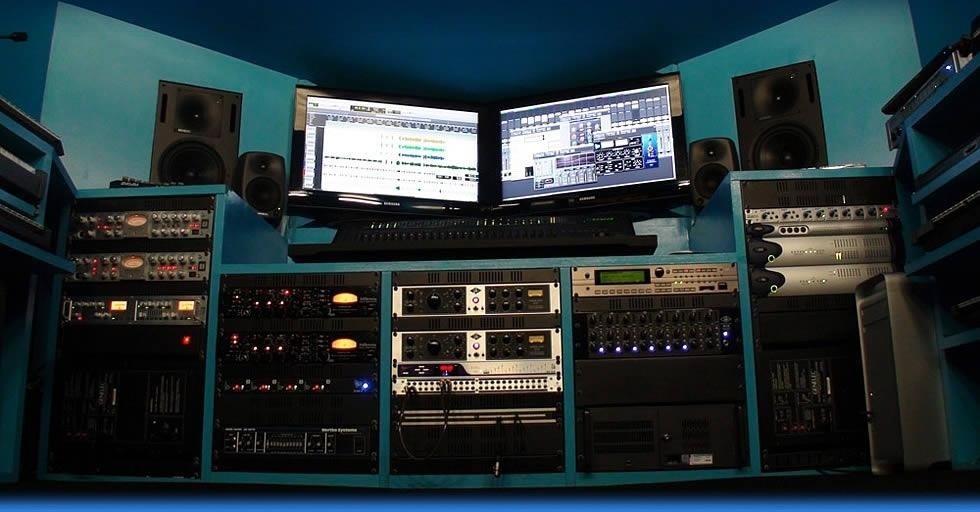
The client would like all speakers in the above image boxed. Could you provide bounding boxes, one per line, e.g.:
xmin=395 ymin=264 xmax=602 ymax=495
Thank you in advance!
xmin=689 ymin=137 xmax=739 ymax=211
xmin=148 ymin=79 xmax=242 ymax=196
xmin=732 ymin=59 xmax=829 ymax=172
xmin=237 ymin=151 xmax=286 ymax=224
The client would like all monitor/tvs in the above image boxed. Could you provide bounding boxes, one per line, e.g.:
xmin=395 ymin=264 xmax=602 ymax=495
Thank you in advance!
xmin=492 ymin=82 xmax=678 ymax=212
xmin=302 ymin=93 xmax=491 ymax=212
